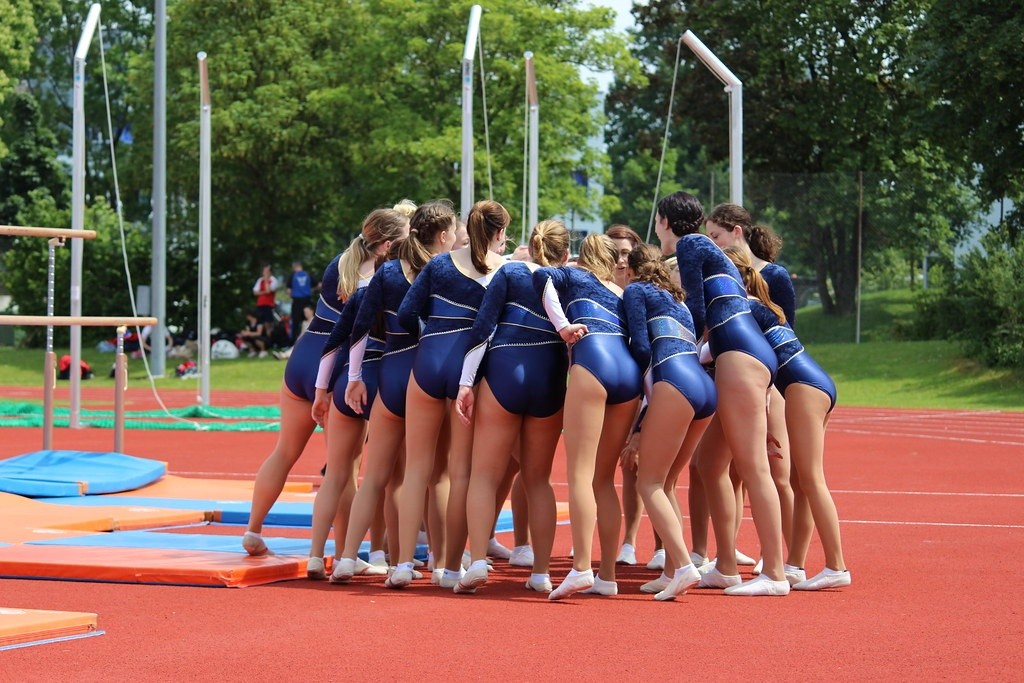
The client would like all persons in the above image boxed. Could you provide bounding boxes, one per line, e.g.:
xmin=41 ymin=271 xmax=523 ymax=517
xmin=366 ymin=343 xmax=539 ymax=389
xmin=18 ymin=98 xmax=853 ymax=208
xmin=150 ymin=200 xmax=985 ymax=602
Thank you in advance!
xmin=59 ymin=319 xmax=200 ymax=382
xmin=243 ymin=191 xmax=851 ymax=600
xmin=237 ymin=262 xmax=322 ymax=360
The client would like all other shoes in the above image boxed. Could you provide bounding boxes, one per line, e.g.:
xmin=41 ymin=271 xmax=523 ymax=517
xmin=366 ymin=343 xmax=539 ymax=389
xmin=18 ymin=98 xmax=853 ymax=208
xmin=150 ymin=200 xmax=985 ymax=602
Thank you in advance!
xmin=575 ymin=577 xmax=617 ymax=596
xmin=724 ymin=576 xmax=790 ymax=595
xmin=368 ymin=557 xmax=390 ymax=572
xmin=616 ymin=544 xmax=637 ymax=565
xmin=647 ymin=548 xmax=665 ymax=569
xmin=487 ymin=539 xmax=511 ymax=558
xmin=639 ymin=575 xmax=689 ymax=595
xmin=258 ymin=352 xmax=267 ymax=359
xmin=754 ymin=558 xmax=763 ymax=573
xmin=785 ymin=568 xmax=806 ymax=586
xmin=331 ymin=557 xmax=386 ymax=575
xmin=307 ymin=558 xmax=325 ymax=578
xmin=735 ymin=548 xmax=755 ymax=565
xmin=654 ymin=565 xmax=701 ymax=600
xmin=389 ymin=567 xmax=421 ymax=578
xmin=247 ymin=351 xmax=254 ymax=357
xmin=547 ymin=570 xmax=594 ymax=599
xmin=330 ymin=559 xmax=354 ymax=583
xmin=508 ymin=551 xmax=534 ymax=566
xmin=385 ymin=553 xmax=424 ymax=567
xmin=690 ymin=553 xmax=710 ymax=568
xmin=384 ymin=568 xmax=409 ymax=587
xmin=427 ymin=551 xmax=495 ymax=593
xmin=273 ymin=351 xmax=286 ymax=360
xmin=526 ymin=579 xmax=552 ymax=591
xmin=694 ymin=557 xmax=742 ymax=588
xmin=791 ymin=568 xmax=852 ymax=591
xmin=242 ymin=536 xmax=275 ymax=556
